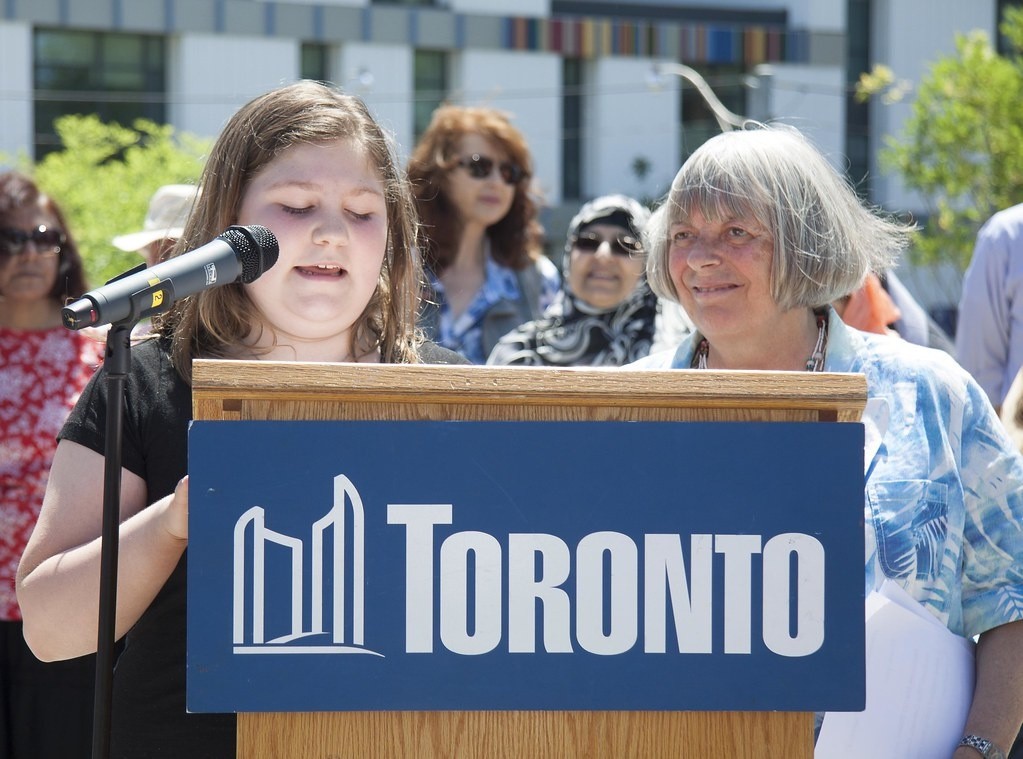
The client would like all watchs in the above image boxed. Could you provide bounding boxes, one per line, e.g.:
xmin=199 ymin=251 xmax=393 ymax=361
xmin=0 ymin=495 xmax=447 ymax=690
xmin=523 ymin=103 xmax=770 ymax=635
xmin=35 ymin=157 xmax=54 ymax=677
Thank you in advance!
xmin=957 ymin=735 xmax=1006 ymax=759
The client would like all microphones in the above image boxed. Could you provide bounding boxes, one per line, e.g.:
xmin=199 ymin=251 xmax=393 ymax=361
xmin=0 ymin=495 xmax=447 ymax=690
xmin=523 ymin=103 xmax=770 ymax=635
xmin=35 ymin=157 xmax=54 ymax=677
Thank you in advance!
xmin=61 ymin=224 xmax=279 ymax=330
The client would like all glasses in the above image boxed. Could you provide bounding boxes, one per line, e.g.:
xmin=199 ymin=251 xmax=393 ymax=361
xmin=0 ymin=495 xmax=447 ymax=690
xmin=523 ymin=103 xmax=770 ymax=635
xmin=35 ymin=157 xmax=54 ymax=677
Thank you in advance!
xmin=0 ymin=227 xmax=63 ymax=256
xmin=442 ymin=156 xmax=522 ymax=185
xmin=576 ymin=236 xmax=637 ymax=257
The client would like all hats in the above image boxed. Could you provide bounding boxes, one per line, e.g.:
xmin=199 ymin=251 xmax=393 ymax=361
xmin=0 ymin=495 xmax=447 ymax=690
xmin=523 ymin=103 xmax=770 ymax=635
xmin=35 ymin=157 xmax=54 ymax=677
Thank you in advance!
xmin=113 ymin=184 xmax=203 ymax=252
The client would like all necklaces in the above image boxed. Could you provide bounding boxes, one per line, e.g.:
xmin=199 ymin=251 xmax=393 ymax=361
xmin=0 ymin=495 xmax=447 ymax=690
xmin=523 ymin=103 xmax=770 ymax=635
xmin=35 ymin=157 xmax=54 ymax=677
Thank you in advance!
xmin=697 ymin=307 xmax=826 ymax=372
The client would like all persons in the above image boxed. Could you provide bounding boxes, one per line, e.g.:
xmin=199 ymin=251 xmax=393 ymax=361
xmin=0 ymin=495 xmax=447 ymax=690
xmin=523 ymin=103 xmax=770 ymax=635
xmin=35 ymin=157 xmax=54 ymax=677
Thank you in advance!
xmin=617 ymin=129 xmax=1023 ymax=759
xmin=484 ymin=194 xmax=696 ymax=368
xmin=956 ymin=203 xmax=1023 ymax=417
xmin=13 ymin=80 xmax=420 ymax=759
xmin=406 ymin=103 xmax=562 ymax=363
xmin=831 ymin=252 xmax=953 ymax=355
xmin=0 ymin=168 xmax=204 ymax=759
